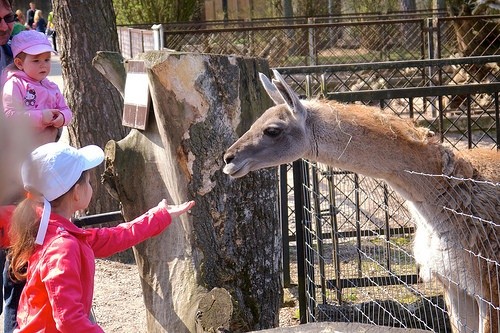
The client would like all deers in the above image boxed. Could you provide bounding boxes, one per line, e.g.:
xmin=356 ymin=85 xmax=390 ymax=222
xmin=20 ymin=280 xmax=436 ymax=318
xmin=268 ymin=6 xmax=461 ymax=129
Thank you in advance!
xmin=223 ymin=68 xmax=500 ymax=333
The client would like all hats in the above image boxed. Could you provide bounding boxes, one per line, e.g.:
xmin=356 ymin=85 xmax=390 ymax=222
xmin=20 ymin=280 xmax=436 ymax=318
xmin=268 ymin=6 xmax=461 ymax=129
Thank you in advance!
xmin=11 ymin=30 xmax=59 ymax=59
xmin=21 ymin=142 xmax=106 ymax=203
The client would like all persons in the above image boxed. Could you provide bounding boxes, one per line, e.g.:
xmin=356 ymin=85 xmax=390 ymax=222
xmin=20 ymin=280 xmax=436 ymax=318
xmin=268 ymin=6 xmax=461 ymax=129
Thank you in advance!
xmin=27 ymin=2 xmax=36 ymax=28
xmin=15 ymin=9 xmax=25 ymax=26
xmin=46 ymin=10 xmax=57 ymax=52
xmin=0 ymin=26 xmax=72 ymax=134
xmin=0 ymin=0 xmax=62 ymax=333
xmin=33 ymin=10 xmax=47 ymax=33
xmin=0 ymin=141 xmax=196 ymax=333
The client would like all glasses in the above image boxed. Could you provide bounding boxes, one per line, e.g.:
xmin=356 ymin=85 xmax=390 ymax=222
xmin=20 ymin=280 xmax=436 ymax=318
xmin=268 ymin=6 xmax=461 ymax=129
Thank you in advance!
xmin=0 ymin=12 xmax=15 ymax=24
